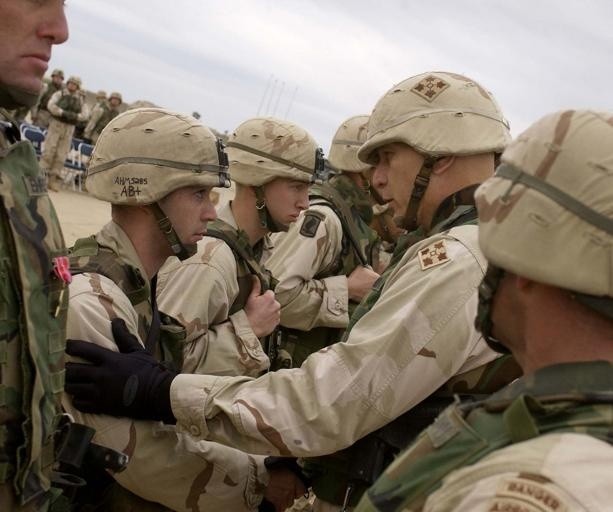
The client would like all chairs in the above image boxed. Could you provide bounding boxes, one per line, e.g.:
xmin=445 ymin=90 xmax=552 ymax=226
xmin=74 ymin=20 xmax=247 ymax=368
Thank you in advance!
xmin=78 ymin=143 xmax=95 ymax=193
xmin=20 ymin=123 xmax=42 ymax=155
xmin=60 ymin=158 xmax=74 ymax=190
xmin=73 ymin=137 xmax=93 ymax=189
xmin=23 ymin=129 xmax=45 ymax=159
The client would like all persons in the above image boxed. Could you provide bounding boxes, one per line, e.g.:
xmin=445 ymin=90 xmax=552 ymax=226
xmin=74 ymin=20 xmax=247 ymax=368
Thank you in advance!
xmin=64 ymin=106 xmax=304 ymax=511
xmin=63 ymin=71 xmax=510 ymax=512
xmin=352 ymin=108 xmax=613 ymax=511
xmin=38 ymin=75 xmax=89 ymax=196
xmin=261 ymin=115 xmax=381 ymax=330
xmin=0 ymin=0 xmax=69 ymax=512
xmin=31 ymin=69 xmax=122 ymax=144
xmin=157 ymin=116 xmax=316 ymax=377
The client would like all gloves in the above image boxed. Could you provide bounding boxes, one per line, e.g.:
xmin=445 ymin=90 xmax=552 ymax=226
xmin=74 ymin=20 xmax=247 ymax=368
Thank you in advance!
xmin=64 ymin=317 xmax=177 ymax=428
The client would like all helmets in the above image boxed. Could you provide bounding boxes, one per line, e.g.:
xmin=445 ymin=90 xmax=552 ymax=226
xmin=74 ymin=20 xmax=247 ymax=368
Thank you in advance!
xmin=86 ymin=108 xmax=232 ymax=206
xmin=51 ymin=69 xmax=64 ymax=80
xmin=222 ymin=117 xmax=317 ymax=187
xmin=108 ymin=92 xmax=122 ymax=103
xmin=96 ymin=90 xmax=107 ymax=98
xmin=66 ymin=74 xmax=82 ymax=89
xmin=473 ymin=109 xmax=613 ymax=298
xmin=356 ymin=69 xmax=513 ymax=166
xmin=327 ymin=115 xmax=369 ymax=173
